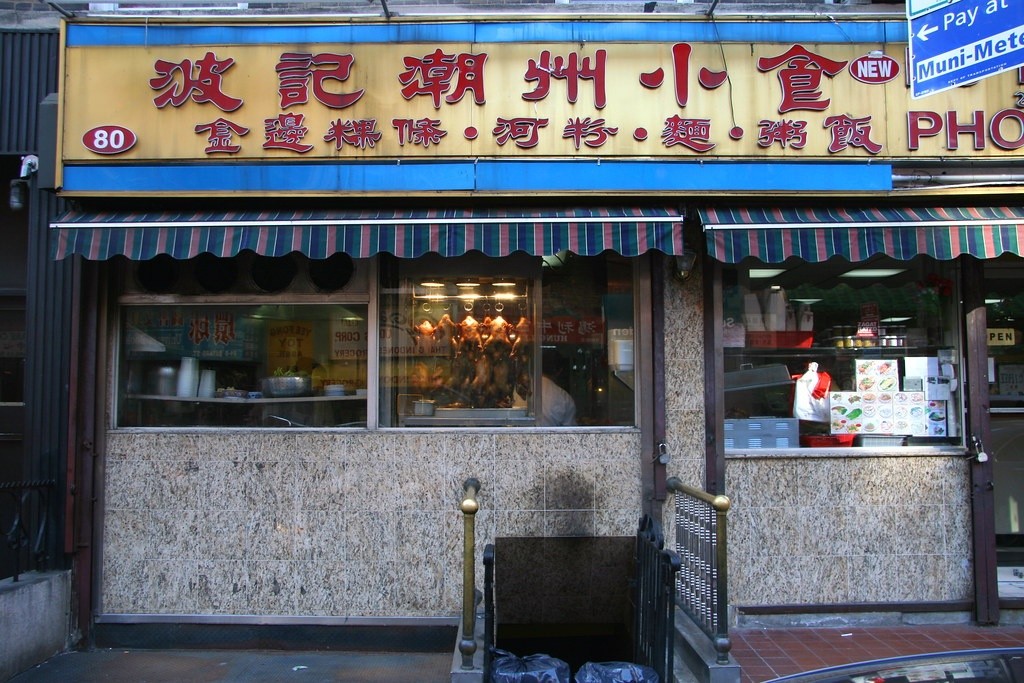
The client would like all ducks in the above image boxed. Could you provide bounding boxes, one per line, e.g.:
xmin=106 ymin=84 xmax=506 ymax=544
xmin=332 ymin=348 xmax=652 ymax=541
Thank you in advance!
xmin=413 ymin=315 xmax=532 ymax=357
xmin=410 ymin=355 xmax=531 ymax=401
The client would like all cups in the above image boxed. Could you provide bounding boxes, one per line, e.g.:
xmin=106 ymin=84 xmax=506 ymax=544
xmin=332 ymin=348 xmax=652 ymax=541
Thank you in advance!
xmin=744 ymin=293 xmax=764 ymax=331
xmin=198 ymin=370 xmax=215 ymax=397
xmin=175 ymin=356 xmax=198 ymax=397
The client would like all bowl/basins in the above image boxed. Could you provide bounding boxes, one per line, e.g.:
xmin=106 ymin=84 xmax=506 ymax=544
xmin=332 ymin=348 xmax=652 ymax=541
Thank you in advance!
xmin=214 ymin=391 xmax=223 ymax=398
xmin=259 ymin=376 xmax=311 ymax=398
xmin=323 ymin=384 xmax=345 ymax=396
xmin=223 ymin=390 xmax=247 ymax=397
xmin=355 ymin=389 xmax=367 ymax=395
xmin=248 ymin=392 xmax=262 ymax=399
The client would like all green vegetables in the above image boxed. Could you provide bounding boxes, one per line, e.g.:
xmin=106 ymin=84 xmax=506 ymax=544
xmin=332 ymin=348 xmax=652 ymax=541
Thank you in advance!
xmin=271 ymin=367 xmax=310 ymax=377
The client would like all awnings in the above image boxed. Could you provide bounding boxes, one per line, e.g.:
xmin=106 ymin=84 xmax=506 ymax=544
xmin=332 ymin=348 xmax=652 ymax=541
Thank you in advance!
xmin=696 ymin=205 xmax=1024 ymax=264
xmin=49 ymin=212 xmax=684 ymax=260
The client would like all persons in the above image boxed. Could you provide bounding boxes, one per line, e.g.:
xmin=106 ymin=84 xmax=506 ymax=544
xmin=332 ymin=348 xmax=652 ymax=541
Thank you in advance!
xmin=804 ymin=358 xmax=843 ymax=433
xmin=506 ymin=350 xmax=576 ymax=426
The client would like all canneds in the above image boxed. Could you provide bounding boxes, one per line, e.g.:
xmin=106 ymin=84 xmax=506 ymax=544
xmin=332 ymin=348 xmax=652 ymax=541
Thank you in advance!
xmin=823 ymin=324 xmax=908 ymax=347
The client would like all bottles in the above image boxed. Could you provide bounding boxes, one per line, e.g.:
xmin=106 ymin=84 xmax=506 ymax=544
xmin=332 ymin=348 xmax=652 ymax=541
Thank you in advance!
xmin=765 ymin=286 xmax=786 ymax=331
